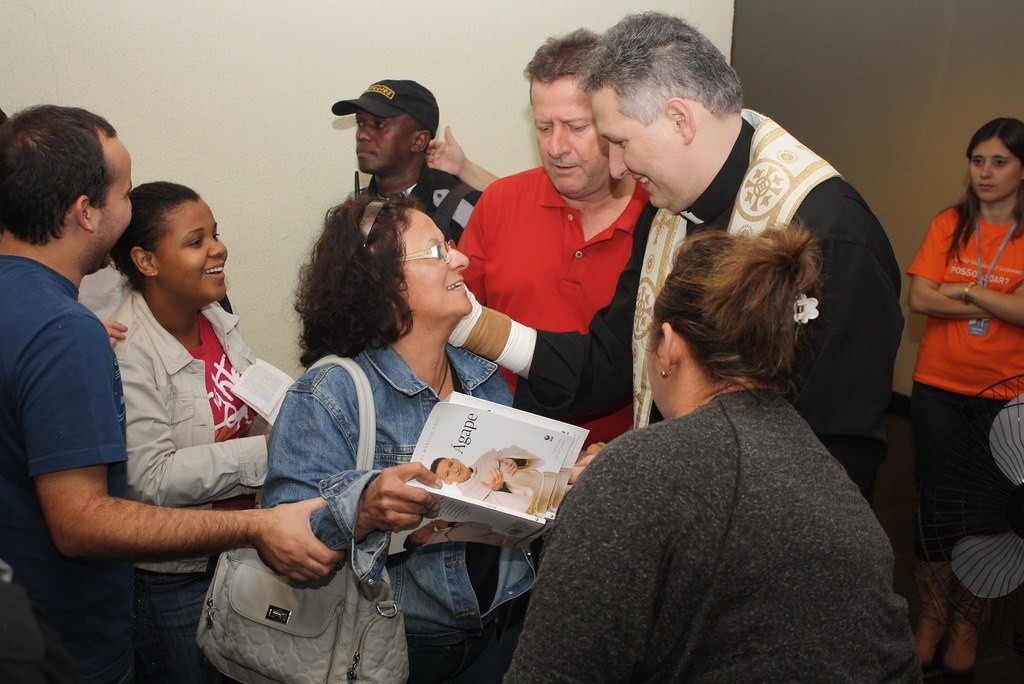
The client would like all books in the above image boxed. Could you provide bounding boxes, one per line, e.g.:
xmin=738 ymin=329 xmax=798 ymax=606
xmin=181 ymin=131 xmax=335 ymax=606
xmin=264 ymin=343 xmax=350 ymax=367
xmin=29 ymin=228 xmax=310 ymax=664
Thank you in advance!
xmin=233 ymin=360 xmax=295 ymax=427
xmin=389 ymin=390 xmax=590 ymax=556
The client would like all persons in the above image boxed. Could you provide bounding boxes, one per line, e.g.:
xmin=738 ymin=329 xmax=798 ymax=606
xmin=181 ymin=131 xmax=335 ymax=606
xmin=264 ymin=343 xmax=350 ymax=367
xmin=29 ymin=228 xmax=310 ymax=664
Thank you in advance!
xmin=259 ymin=200 xmax=607 ymax=684
xmin=904 ymin=118 xmax=1024 ymax=684
xmin=0 ymin=106 xmax=345 ymax=684
xmin=432 ymin=446 xmax=558 ymax=515
xmin=450 ymin=12 xmax=902 ymax=517
xmin=334 ymin=76 xmax=498 ymax=252
xmin=457 ymin=25 xmax=653 ymax=451
xmin=975 ymin=318 xmax=982 ymax=328
xmin=403 ymin=520 xmax=524 ymax=551
xmin=503 ymin=226 xmax=920 ymax=684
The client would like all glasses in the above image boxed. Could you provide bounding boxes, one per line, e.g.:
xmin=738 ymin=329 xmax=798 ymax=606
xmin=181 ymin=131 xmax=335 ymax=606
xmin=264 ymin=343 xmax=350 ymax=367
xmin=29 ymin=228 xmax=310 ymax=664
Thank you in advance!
xmin=396 ymin=239 xmax=456 ymax=265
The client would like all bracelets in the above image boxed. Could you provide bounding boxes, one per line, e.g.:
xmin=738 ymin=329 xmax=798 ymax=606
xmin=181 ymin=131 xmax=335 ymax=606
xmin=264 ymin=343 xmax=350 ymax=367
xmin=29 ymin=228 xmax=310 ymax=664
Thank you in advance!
xmin=963 ymin=282 xmax=975 ymax=304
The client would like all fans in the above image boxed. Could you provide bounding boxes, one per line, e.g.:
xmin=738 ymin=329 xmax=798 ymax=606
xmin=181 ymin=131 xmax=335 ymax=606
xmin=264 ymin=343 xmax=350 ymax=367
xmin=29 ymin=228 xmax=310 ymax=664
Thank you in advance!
xmin=917 ymin=374 xmax=1024 ymax=663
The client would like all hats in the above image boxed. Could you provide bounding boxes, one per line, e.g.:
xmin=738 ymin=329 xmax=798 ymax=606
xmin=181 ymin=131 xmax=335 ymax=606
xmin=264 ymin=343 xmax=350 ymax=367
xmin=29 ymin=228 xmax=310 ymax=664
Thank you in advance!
xmin=332 ymin=79 xmax=439 ymax=139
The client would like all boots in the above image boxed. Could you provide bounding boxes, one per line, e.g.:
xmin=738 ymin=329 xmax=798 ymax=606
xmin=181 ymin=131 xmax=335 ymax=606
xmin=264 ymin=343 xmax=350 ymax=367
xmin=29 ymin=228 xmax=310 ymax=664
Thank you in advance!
xmin=943 ymin=575 xmax=981 ymax=672
xmin=912 ymin=556 xmax=950 ymax=665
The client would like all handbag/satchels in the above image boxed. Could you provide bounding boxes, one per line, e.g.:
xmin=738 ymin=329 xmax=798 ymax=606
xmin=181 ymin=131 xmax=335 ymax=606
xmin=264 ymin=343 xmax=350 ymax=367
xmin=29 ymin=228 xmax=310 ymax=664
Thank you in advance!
xmin=194 ymin=355 xmax=409 ymax=684
